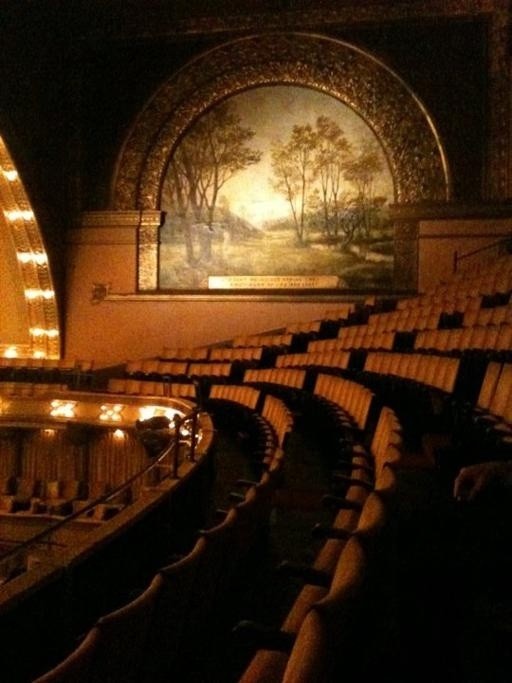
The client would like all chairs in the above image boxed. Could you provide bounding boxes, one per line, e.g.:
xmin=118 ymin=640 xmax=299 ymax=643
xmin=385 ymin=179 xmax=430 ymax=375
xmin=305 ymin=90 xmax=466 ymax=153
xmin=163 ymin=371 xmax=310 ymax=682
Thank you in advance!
xmin=231 ymin=252 xmax=512 ymax=350
xmin=0 ymin=346 xmax=512 ymax=683
